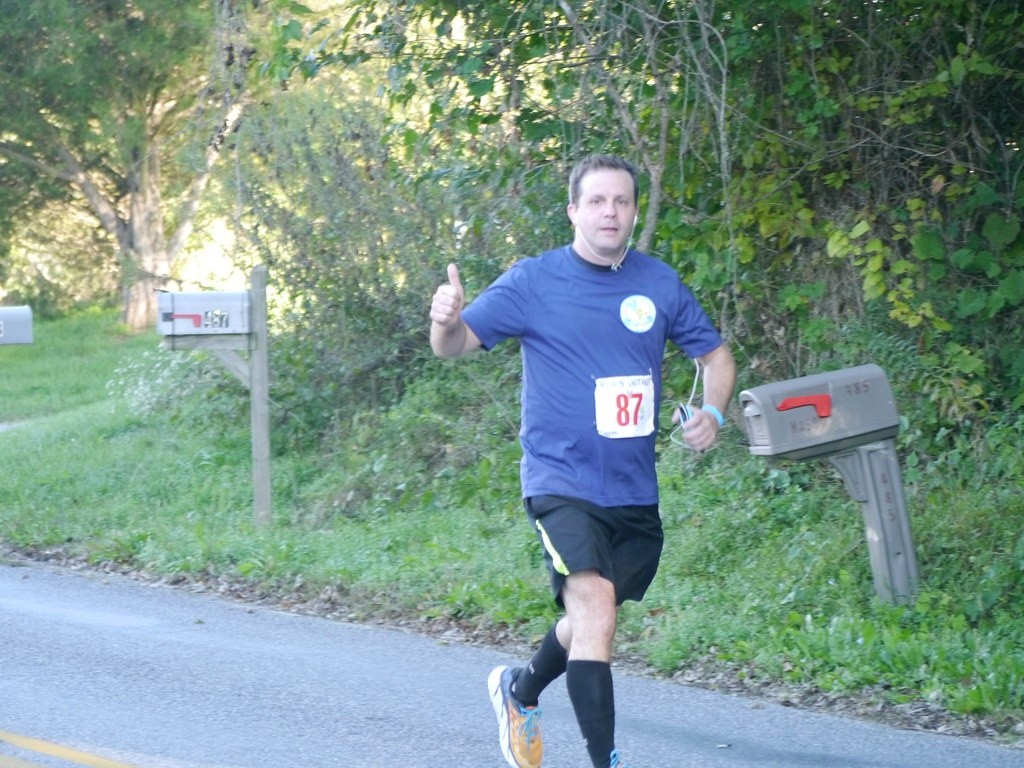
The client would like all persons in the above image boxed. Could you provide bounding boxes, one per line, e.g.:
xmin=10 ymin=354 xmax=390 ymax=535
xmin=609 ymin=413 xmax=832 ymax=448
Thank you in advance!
xmin=429 ymin=154 xmax=737 ymax=768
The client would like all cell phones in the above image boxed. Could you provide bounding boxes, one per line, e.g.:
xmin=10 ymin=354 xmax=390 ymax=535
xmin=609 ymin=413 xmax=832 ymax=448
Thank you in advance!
xmin=678 ymin=403 xmax=689 ymax=422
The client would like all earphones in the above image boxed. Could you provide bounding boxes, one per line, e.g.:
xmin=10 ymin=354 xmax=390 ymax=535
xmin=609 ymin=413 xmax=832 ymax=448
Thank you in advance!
xmin=573 ymin=213 xmax=578 ymax=224
xmin=633 ymin=214 xmax=638 ymax=228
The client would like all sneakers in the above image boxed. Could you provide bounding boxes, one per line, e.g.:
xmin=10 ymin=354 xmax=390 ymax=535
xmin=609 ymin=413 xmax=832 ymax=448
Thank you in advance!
xmin=488 ymin=664 xmax=543 ymax=768
xmin=608 ymin=749 xmax=629 ymax=768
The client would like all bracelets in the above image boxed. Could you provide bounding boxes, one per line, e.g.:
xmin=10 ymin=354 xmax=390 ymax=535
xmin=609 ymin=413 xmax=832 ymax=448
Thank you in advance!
xmin=702 ymin=405 xmax=725 ymax=426
